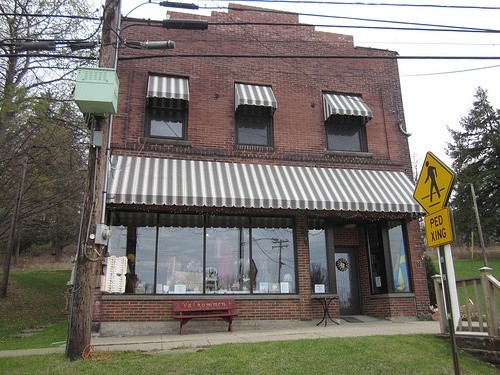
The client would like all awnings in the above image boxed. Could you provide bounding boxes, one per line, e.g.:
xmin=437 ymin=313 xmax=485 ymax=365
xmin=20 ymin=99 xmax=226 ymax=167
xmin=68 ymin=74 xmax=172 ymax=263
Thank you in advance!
xmin=105 ymin=154 xmax=428 ymax=220
xmin=146 ymin=74 xmax=190 ymax=104
xmin=322 ymin=91 xmax=373 ymax=127
xmin=235 ymin=82 xmax=279 ymax=117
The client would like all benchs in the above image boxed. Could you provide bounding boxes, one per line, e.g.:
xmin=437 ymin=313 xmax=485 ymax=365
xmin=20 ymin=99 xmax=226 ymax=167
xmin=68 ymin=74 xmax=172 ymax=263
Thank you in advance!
xmin=174 ymin=298 xmax=238 ymax=336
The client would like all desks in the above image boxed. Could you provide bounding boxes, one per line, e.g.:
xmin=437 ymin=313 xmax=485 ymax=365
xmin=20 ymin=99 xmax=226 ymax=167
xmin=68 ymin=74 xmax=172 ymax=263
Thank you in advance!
xmin=310 ymin=295 xmax=340 ymax=327
